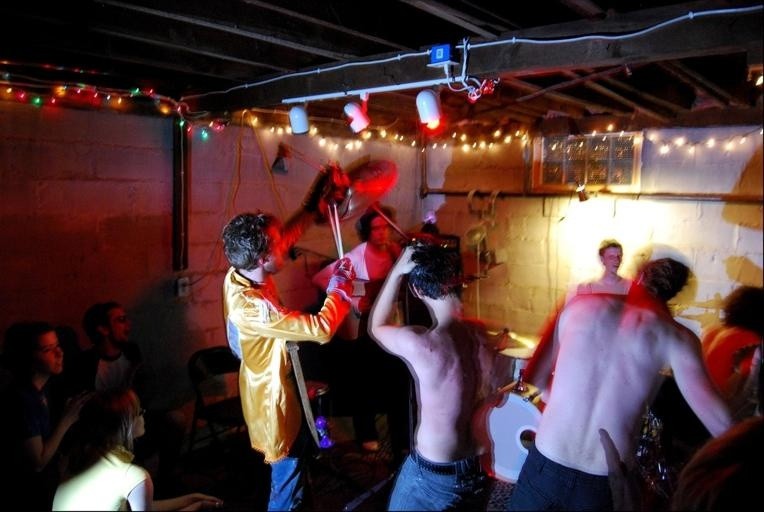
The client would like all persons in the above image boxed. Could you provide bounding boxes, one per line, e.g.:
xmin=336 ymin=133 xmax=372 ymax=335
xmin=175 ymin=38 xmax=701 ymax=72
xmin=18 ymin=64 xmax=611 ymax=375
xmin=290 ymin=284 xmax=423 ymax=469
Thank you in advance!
xmin=503 ymin=246 xmax=736 ymax=511
xmin=669 ymin=285 xmax=763 ymax=469
xmin=51 ymin=383 xmax=223 ymax=512
xmin=296 ymin=259 xmax=409 ymax=452
xmin=349 ymin=212 xmax=397 ymax=341
xmin=220 ymin=213 xmax=357 ymax=512
xmin=74 ymin=303 xmax=146 ymax=466
xmin=365 ymin=242 xmax=490 ymax=511
xmin=1 ymin=320 xmax=96 ymax=511
xmin=575 ymin=240 xmax=629 ymax=294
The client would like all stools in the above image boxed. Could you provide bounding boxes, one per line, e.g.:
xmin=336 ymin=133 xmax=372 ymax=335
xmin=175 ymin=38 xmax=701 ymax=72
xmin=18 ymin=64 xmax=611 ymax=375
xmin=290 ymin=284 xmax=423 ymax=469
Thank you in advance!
xmin=305 ymin=379 xmax=332 ymax=416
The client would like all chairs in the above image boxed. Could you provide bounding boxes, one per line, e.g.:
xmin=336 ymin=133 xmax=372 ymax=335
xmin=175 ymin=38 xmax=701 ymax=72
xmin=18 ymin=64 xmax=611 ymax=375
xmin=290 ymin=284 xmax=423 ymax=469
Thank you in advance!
xmin=188 ymin=345 xmax=246 ymax=451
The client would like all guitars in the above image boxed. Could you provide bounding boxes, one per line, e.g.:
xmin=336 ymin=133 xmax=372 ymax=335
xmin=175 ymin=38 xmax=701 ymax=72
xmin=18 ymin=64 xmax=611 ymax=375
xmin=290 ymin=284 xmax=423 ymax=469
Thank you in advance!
xmin=632 ymin=404 xmax=675 ymax=504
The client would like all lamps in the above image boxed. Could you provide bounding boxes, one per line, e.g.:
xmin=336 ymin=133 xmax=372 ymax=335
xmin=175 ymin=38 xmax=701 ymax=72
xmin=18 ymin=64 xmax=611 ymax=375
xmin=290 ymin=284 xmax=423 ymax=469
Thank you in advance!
xmin=281 ymin=75 xmax=470 ymax=136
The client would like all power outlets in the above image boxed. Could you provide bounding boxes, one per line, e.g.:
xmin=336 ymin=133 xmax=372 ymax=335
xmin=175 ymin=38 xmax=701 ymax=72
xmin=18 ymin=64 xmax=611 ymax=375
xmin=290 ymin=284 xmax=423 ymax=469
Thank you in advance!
xmin=176 ymin=277 xmax=190 ymax=298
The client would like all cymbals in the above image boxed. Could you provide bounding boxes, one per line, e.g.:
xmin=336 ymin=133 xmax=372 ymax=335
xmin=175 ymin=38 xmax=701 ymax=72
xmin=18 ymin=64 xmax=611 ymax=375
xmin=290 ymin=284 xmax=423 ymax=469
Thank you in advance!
xmin=480 ymin=329 xmax=539 ymax=360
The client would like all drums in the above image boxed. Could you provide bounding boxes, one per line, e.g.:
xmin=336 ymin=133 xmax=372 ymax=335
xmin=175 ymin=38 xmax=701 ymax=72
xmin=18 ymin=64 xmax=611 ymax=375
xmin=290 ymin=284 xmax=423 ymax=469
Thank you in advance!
xmin=473 ymin=380 xmax=548 ymax=487
